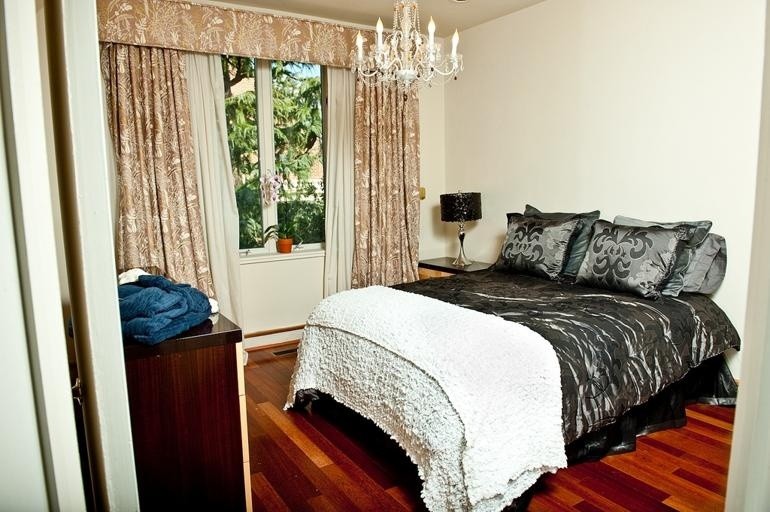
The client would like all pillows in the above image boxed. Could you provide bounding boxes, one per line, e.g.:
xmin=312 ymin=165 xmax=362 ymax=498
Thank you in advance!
xmin=494 ymin=202 xmax=728 ymax=302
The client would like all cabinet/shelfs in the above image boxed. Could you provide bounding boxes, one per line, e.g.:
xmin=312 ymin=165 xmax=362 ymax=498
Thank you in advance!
xmin=116 ymin=265 xmax=254 ymax=512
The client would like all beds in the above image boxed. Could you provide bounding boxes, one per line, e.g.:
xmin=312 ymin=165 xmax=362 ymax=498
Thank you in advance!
xmin=305 ymin=270 xmax=718 ymax=512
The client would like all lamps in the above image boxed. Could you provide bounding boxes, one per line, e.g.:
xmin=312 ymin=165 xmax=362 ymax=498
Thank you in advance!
xmin=439 ymin=190 xmax=483 ymax=266
xmin=349 ymin=0 xmax=465 ymax=98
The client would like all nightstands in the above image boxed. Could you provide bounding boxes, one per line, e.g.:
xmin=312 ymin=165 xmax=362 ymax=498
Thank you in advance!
xmin=419 ymin=257 xmax=493 ymax=280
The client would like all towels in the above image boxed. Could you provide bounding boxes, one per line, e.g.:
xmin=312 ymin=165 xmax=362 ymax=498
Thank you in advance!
xmin=118 ymin=267 xmax=219 ymax=346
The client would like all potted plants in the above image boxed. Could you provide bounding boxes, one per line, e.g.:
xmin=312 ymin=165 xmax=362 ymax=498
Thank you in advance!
xmin=262 ymin=221 xmax=302 ymax=254
